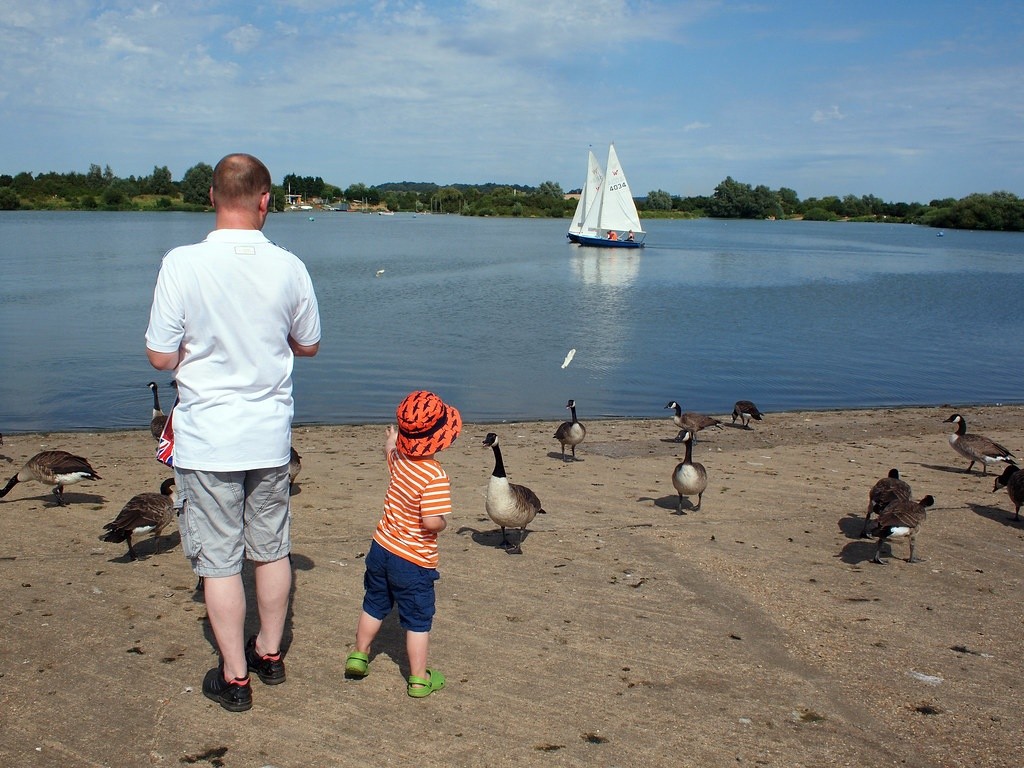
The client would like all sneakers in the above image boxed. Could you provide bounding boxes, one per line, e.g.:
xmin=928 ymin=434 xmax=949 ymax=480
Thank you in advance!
xmin=202 ymin=667 xmax=253 ymax=713
xmin=245 ymin=634 xmax=287 ymax=685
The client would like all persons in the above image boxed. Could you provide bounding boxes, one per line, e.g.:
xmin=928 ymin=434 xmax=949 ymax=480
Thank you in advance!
xmin=346 ymin=390 xmax=462 ymax=697
xmin=607 ymin=230 xmax=617 ymax=241
xmin=628 ymin=230 xmax=634 ymax=239
xmin=145 ymin=153 xmax=321 ymax=710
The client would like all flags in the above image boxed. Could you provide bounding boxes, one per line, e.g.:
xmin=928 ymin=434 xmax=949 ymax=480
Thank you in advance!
xmin=156 ymin=395 xmax=178 ymax=470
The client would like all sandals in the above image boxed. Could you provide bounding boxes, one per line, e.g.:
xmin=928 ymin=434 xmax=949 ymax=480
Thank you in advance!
xmin=344 ymin=652 xmax=369 ymax=677
xmin=408 ymin=668 xmax=446 ymax=698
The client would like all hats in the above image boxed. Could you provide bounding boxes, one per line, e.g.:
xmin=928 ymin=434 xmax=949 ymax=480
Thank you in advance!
xmin=395 ymin=390 xmax=462 ymax=457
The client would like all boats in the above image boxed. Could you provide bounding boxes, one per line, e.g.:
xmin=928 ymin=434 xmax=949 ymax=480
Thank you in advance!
xmin=936 ymin=231 xmax=944 ymax=237
xmin=281 ymin=180 xmax=439 ymax=222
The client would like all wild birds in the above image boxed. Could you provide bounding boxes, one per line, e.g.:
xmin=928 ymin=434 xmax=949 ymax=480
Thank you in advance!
xmin=670 ymin=429 xmax=708 ymax=515
xmin=146 ymin=381 xmax=168 ymax=450
xmin=664 ymin=401 xmax=724 ymax=446
xmin=553 ymin=399 xmax=586 ymax=463
xmin=859 ymin=469 xmax=935 ymax=565
xmin=732 ymin=401 xmax=765 ymax=427
xmin=0 ymin=451 xmax=102 ymax=508
xmin=942 ymin=414 xmax=1017 ymax=474
xmin=97 ymin=477 xmax=176 ymax=561
xmin=991 ymin=464 xmax=1024 ymax=521
xmin=482 ymin=433 xmax=546 ymax=555
xmin=289 ymin=447 xmax=303 ymax=493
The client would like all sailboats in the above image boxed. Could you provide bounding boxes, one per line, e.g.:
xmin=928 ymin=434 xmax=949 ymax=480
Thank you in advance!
xmin=567 ymin=140 xmax=647 ymax=247
